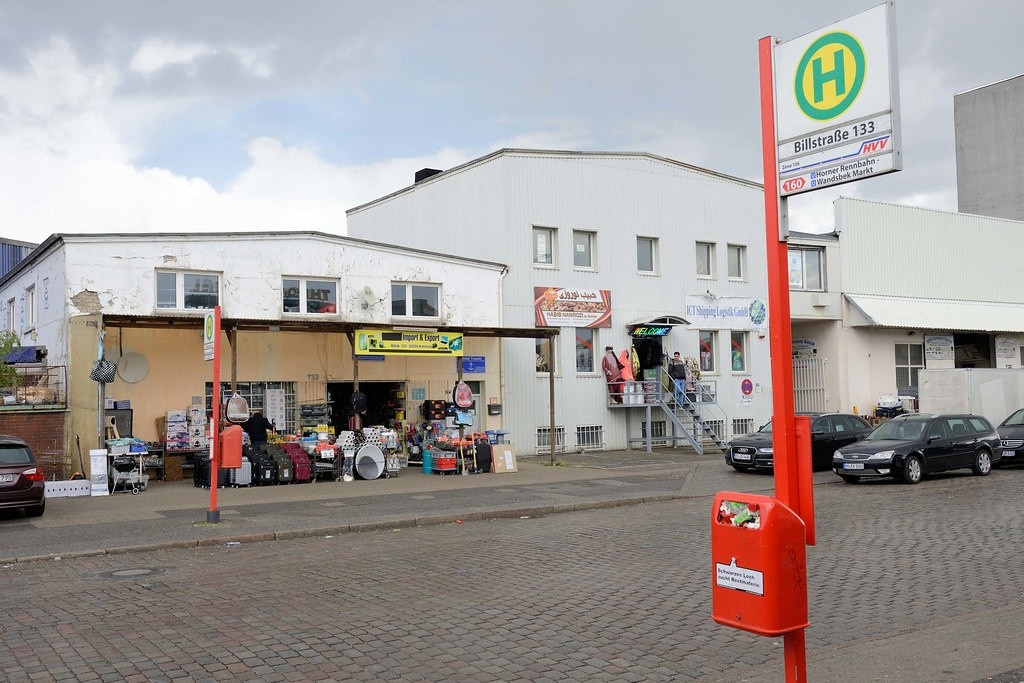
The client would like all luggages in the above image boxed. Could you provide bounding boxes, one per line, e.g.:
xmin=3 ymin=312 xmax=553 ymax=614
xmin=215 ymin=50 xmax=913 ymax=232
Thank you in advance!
xmin=193 ymin=443 xmax=311 ymax=488
xmin=472 ymin=441 xmax=492 ymax=473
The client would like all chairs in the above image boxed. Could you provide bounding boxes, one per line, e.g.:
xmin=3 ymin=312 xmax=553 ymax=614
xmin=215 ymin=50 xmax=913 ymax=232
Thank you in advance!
xmin=952 ymin=424 xmax=966 ymax=434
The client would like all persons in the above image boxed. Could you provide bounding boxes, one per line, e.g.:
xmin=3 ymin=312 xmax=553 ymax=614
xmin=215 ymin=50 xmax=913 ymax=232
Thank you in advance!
xmin=239 ymin=411 xmax=276 ymax=450
xmin=668 ymin=352 xmax=686 ymax=409
xmin=602 ymin=346 xmax=634 ymax=404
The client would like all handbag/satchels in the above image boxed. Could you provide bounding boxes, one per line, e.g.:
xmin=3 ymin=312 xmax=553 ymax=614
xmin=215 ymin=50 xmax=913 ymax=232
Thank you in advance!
xmin=351 ymin=392 xmax=367 ymax=413
xmin=90 ymin=357 xmax=117 ymax=383
xmin=226 ymin=392 xmax=250 ymax=423
xmin=453 ymin=379 xmax=473 ymax=408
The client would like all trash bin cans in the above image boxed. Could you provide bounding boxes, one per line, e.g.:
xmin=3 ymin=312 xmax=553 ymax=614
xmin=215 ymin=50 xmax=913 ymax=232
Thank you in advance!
xmin=219 ymin=425 xmax=242 ymax=469
xmin=712 ymin=492 xmax=810 ymax=637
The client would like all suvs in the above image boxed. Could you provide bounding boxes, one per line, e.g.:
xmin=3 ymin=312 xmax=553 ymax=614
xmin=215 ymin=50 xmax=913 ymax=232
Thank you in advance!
xmin=831 ymin=411 xmax=1003 ymax=485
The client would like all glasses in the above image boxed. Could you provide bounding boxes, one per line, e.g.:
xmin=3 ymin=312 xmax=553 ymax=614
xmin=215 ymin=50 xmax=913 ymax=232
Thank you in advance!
xmin=674 ymin=356 xmax=678 ymax=357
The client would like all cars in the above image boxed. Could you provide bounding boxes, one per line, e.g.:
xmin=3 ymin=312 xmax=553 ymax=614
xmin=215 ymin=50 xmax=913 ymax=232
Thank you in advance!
xmin=0 ymin=435 xmax=46 ymax=517
xmin=723 ymin=411 xmax=875 ymax=472
xmin=992 ymin=407 xmax=1024 ymax=468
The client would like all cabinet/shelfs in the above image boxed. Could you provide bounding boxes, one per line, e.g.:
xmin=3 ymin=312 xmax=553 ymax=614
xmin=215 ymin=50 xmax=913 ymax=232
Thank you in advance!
xmin=105 ymin=409 xmax=133 ymax=441
xmin=389 ymin=419 xmax=407 ymax=455
xmin=308 ymin=452 xmax=342 ymax=484
xmin=107 ymin=451 xmax=149 ymax=488
xmin=135 ymin=443 xmax=210 ymax=481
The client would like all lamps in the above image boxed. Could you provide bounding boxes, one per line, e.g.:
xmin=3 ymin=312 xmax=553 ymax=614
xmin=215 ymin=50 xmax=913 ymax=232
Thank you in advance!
xmin=908 ymin=330 xmax=916 ymax=336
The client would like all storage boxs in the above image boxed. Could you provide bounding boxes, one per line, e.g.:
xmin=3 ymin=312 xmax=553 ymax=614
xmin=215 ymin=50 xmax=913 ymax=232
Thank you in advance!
xmin=878 ymin=395 xmax=902 ymax=408
xmin=107 ymin=444 xmax=130 ymax=453
xmin=113 ymin=400 xmax=130 ymax=410
xmin=623 ymin=384 xmax=645 ymax=406
xmin=299 ymin=424 xmax=335 ymax=450
xmin=431 ymin=452 xmax=457 ymax=470
xmin=166 ymin=406 xmax=209 ymax=451
xmin=687 ymin=386 xmax=713 ymax=401
xmin=470 ymin=429 xmax=519 ymax=474
xmin=104 ymin=398 xmax=114 ymax=410
xmin=262 ymin=389 xmax=286 ymax=431
xmin=131 ymin=443 xmax=146 ymax=452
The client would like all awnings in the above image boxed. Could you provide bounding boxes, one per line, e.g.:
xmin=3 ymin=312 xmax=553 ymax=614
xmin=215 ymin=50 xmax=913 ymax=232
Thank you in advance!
xmin=845 ymin=294 xmax=1024 ymax=332
